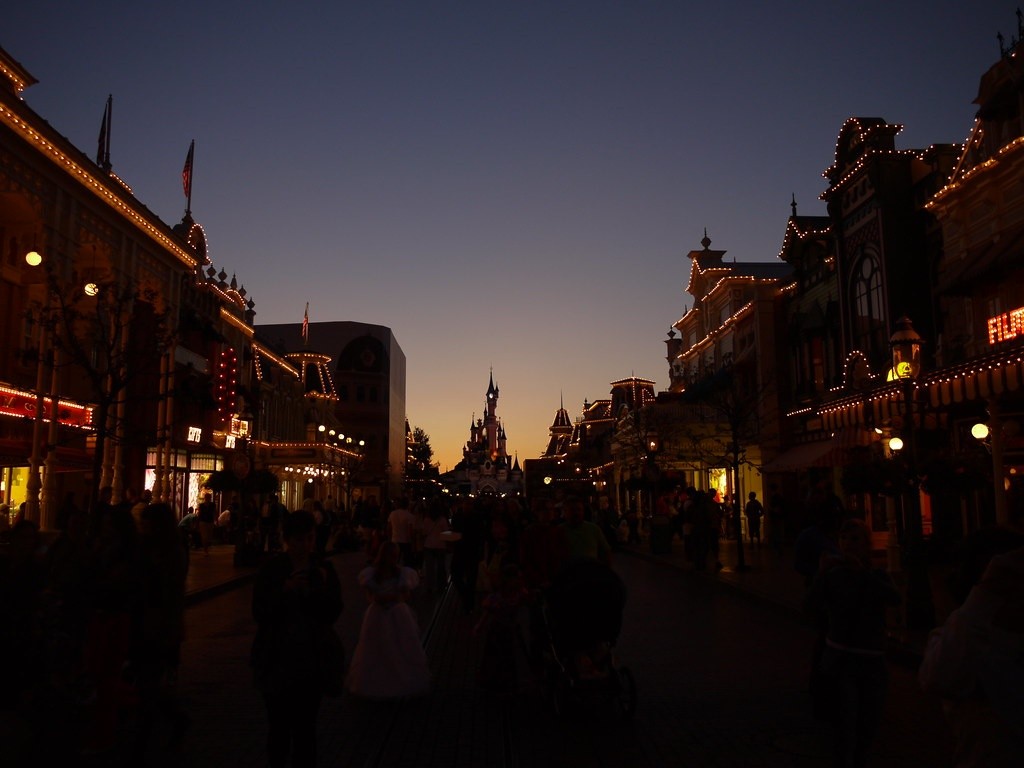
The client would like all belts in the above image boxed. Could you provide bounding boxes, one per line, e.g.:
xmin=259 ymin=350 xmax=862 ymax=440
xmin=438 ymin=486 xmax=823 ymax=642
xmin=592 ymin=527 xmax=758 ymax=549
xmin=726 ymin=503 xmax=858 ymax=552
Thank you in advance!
xmin=825 ymin=636 xmax=885 ymax=657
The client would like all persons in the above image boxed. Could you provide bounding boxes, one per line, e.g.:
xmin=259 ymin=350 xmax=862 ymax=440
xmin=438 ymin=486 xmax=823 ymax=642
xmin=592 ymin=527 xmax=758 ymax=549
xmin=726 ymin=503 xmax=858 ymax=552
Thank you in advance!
xmin=198 ymin=487 xmax=733 ymax=768
xmin=1 ymin=487 xmax=192 ymax=754
xmin=919 ymin=528 xmax=1024 ymax=767
xmin=792 ymin=506 xmax=891 ymax=768
xmin=746 ymin=493 xmax=764 ymax=549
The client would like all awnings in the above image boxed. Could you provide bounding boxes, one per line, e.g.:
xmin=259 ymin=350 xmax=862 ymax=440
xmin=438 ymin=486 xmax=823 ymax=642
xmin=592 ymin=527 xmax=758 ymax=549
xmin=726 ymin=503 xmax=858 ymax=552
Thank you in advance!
xmin=761 ymin=440 xmax=833 ymax=473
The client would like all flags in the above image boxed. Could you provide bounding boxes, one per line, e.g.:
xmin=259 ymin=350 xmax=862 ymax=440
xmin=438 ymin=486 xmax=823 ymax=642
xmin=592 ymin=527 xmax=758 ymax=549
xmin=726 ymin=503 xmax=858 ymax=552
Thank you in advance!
xmin=97 ymin=99 xmax=112 ymax=165
xmin=182 ymin=143 xmax=193 ymax=197
